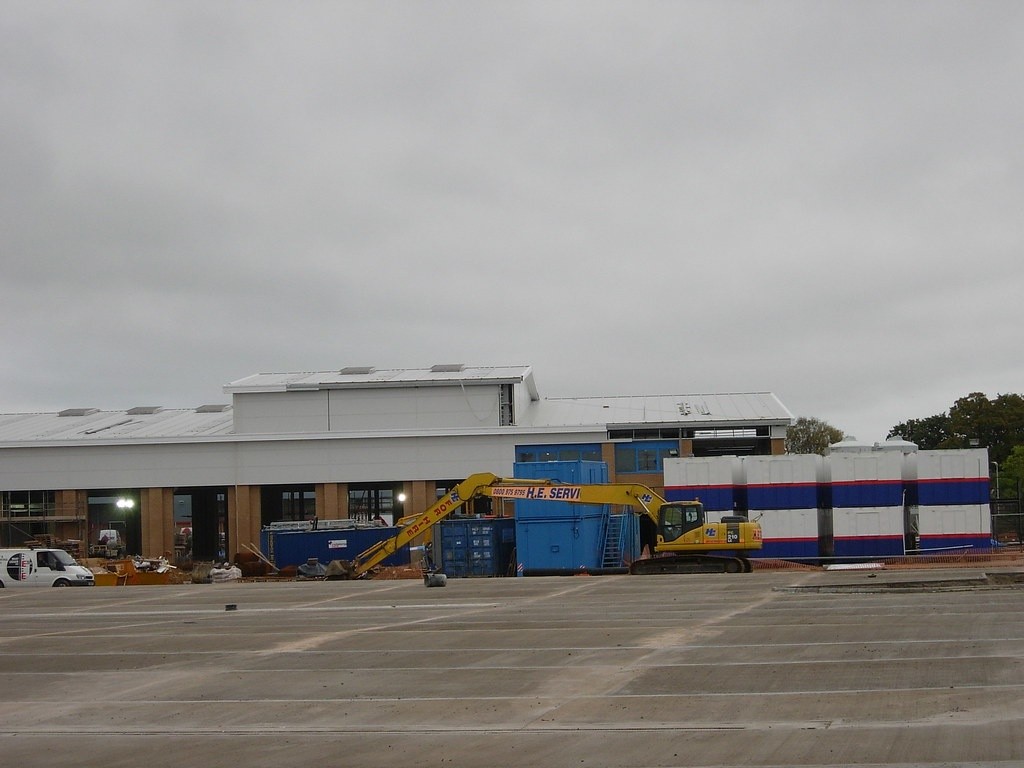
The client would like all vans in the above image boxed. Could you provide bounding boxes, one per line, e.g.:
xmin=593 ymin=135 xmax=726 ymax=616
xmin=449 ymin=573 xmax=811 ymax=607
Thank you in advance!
xmin=1 ymin=546 xmax=98 ymax=587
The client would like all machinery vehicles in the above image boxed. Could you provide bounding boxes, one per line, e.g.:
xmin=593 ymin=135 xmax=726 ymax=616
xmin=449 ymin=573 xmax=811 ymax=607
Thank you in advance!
xmin=320 ymin=471 xmax=766 ymax=573
